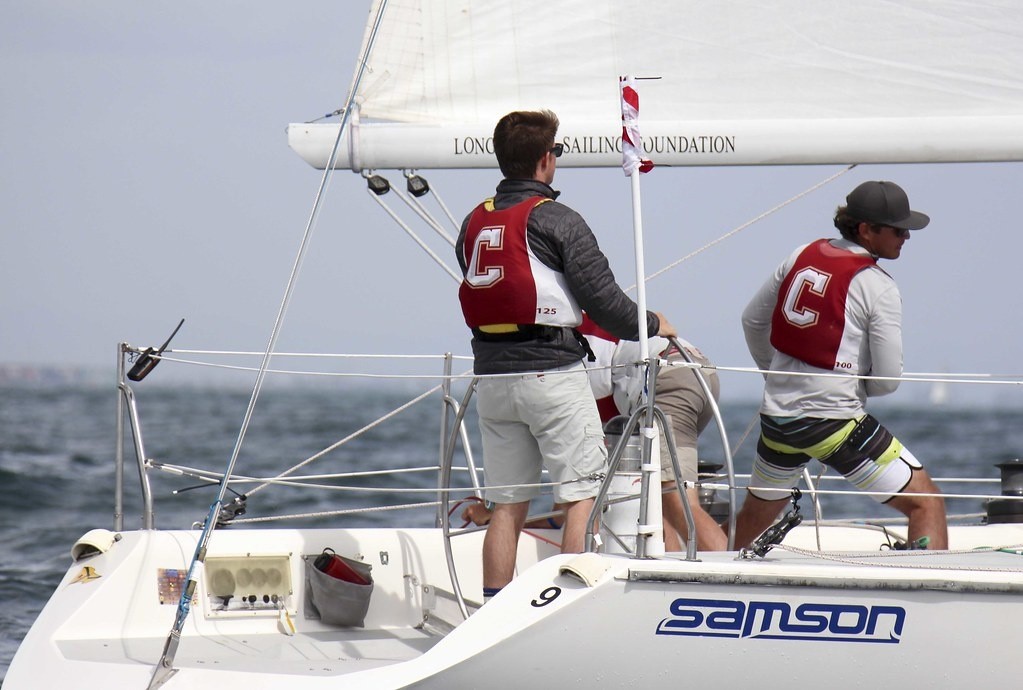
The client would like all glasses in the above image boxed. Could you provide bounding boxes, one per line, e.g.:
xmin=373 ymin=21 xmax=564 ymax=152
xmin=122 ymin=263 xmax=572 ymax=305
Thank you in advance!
xmin=537 ymin=143 xmax=563 ymax=157
xmin=875 ymin=223 xmax=906 ymax=237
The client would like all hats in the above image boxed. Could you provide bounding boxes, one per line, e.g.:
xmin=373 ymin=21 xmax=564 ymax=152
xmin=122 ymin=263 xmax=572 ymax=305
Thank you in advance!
xmin=846 ymin=181 xmax=930 ymax=230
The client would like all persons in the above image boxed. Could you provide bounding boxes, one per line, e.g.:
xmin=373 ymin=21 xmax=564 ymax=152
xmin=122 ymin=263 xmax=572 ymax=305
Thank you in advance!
xmin=455 ymin=112 xmax=678 ymax=604
xmin=732 ymin=182 xmax=948 ymax=550
xmin=463 ymin=314 xmax=728 ymax=552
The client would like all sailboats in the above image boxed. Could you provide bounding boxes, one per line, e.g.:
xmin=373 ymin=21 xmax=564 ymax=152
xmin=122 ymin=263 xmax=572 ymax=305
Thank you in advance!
xmin=0 ymin=0 xmax=1023 ymax=690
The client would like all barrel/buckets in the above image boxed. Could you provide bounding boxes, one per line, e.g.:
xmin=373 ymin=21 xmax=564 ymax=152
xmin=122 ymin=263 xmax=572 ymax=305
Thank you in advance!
xmin=603 ymin=414 xmax=642 ymax=472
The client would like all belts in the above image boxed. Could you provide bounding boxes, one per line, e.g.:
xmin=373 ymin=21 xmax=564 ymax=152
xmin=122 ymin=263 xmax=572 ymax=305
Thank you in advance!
xmin=659 ymin=347 xmax=689 ymax=358
